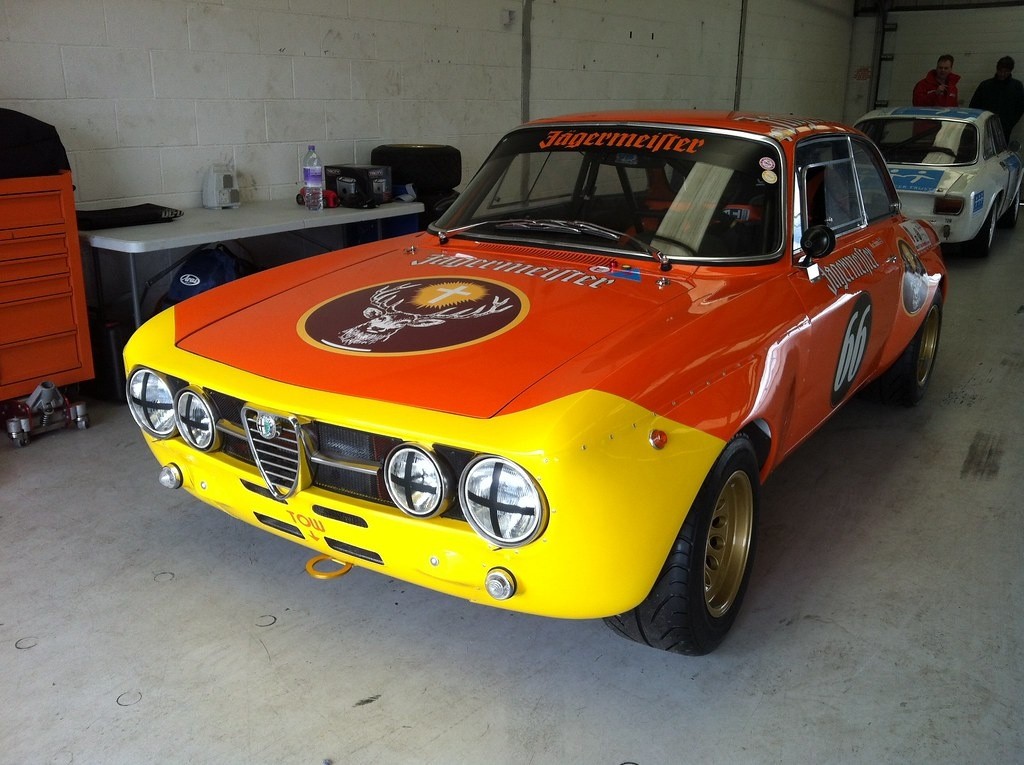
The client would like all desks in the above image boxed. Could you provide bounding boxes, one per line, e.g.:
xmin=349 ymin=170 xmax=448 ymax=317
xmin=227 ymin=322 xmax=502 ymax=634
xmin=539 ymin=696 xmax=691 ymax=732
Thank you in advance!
xmin=76 ymin=198 xmax=425 ymax=403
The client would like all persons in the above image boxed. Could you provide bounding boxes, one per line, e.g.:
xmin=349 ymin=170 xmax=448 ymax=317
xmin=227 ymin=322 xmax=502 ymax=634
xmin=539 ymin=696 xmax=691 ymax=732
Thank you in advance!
xmin=912 ymin=55 xmax=961 ymax=137
xmin=970 ymin=58 xmax=1024 ymax=147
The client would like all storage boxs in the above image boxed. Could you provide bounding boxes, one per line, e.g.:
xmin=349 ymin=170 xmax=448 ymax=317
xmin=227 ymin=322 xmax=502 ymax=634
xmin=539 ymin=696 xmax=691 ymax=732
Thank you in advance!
xmin=324 ymin=163 xmax=392 ymax=204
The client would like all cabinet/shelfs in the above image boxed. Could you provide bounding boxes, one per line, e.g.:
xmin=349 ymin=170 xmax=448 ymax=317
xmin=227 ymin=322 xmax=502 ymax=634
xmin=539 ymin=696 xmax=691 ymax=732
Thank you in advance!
xmin=0 ymin=170 xmax=95 ymax=408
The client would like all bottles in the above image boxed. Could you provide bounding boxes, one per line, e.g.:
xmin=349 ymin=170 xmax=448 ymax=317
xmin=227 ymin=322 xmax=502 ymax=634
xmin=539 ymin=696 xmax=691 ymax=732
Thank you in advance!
xmin=303 ymin=145 xmax=323 ymax=211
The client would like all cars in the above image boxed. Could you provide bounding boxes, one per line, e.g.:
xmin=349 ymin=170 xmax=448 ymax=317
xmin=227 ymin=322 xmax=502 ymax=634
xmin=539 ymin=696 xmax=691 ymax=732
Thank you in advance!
xmin=121 ymin=111 xmax=952 ymax=657
xmin=844 ymin=106 xmax=1024 ymax=258
